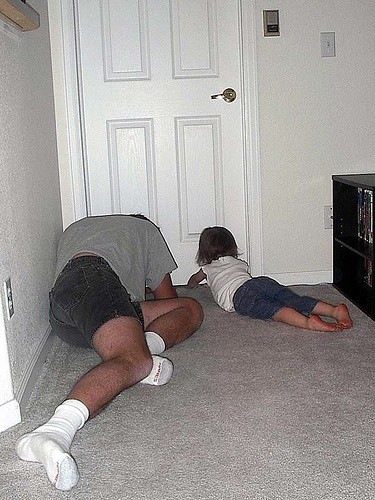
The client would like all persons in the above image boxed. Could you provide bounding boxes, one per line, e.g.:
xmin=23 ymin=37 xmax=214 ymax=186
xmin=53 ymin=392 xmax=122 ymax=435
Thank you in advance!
xmin=15 ymin=213 xmax=203 ymax=490
xmin=188 ymin=227 xmax=353 ymax=331
